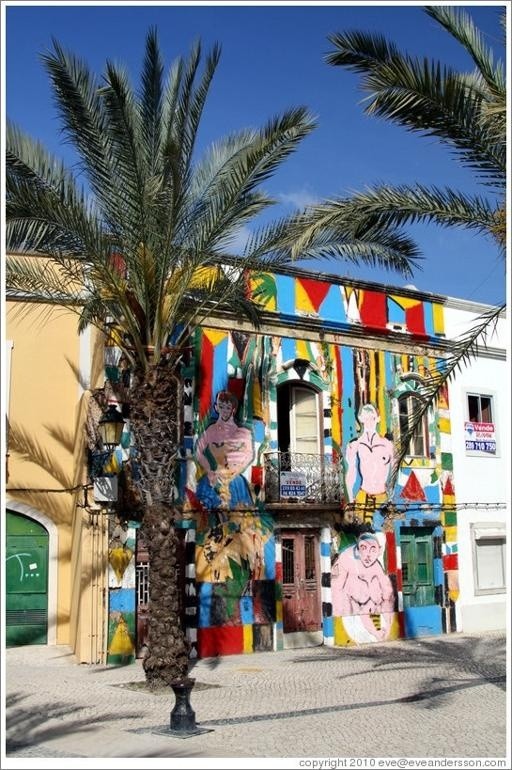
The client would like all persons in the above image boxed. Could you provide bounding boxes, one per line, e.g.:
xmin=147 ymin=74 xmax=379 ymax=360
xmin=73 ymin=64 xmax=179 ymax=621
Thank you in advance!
xmin=195 ymin=390 xmax=254 ymax=510
xmin=345 ymin=404 xmax=395 ymax=529
xmin=329 ymin=533 xmax=394 ymax=614
